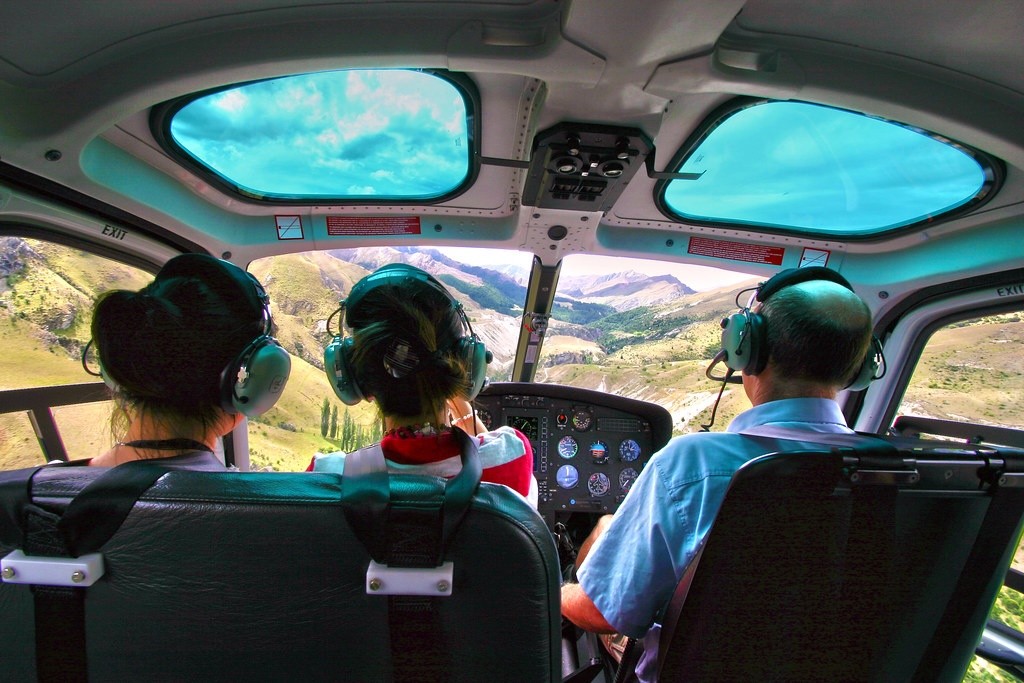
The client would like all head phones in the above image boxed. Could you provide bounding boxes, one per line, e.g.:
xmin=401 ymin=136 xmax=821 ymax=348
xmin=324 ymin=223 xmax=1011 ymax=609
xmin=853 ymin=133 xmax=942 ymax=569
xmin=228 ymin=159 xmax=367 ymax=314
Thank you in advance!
xmin=324 ymin=268 xmax=493 ymax=407
xmin=97 ymin=252 xmax=291 ymax=418
xmin=722 ymin=266 xmax=886 ymax=391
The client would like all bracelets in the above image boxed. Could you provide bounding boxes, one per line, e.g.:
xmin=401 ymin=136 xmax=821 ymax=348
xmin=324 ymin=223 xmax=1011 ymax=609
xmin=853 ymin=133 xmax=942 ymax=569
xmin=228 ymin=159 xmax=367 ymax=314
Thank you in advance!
xmin=450 ymin=409 xmax=478 ymax=425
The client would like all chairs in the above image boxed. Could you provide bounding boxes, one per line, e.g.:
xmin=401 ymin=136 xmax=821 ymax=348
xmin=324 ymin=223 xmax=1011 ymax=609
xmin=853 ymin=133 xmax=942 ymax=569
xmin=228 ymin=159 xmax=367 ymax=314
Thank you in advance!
xmin=633 ymin=449 xmax=1024 ymax=683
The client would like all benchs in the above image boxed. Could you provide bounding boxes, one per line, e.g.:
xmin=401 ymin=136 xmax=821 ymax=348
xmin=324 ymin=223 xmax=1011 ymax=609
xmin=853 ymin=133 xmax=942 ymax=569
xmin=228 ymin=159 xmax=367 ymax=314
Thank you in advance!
xmin=0 ymin=468 xmax=562 ymax=683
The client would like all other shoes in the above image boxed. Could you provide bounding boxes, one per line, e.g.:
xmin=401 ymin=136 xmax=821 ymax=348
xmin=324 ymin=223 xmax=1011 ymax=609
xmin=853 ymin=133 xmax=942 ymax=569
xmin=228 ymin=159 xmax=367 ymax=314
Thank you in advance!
xmin=561 ymin=564 xmax=577 ymax=584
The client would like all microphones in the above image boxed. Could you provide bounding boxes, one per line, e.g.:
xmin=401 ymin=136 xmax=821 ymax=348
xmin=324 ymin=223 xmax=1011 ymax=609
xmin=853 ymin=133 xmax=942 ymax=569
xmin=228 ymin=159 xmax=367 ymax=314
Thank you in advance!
xmin=706 ymin=350 xmax=743 ymax=385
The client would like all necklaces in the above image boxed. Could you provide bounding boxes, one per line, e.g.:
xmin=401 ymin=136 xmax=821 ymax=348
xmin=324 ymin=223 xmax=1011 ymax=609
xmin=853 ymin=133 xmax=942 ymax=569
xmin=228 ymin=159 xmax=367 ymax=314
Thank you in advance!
xmin=114 ymin=437 xmax=214 ymax=450
xmin=384 ymin=422 xmax=452 ymax=437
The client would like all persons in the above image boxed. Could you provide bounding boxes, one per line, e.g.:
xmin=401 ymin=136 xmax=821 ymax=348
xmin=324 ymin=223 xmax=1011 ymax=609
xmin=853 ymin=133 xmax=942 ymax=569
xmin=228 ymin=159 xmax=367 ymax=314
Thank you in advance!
xmin=302 ymin=263 xmax=533 ymax=506
xmin=553 ymin=266 xmax=877 ymax=683
xmin=47 ymin=253 xmax=291 ymax=474
